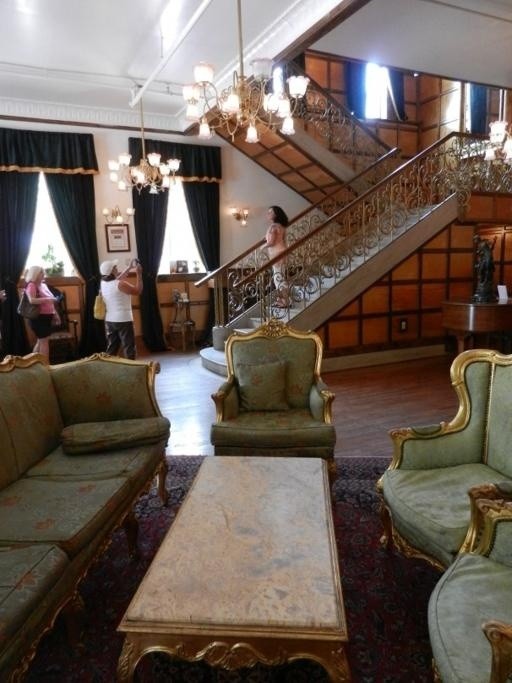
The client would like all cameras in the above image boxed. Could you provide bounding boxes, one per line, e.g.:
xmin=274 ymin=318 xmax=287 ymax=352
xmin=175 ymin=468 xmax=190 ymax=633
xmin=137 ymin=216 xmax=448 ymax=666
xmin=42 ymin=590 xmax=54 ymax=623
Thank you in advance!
xmin=134 ymin=258 xmax=139 ymax=265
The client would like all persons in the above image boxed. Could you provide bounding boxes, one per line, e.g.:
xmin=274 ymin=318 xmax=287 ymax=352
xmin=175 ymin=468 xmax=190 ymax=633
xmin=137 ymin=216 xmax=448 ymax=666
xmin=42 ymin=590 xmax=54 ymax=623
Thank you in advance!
xmin=99 ymin=259 xmax=144 ymax=361
xmin=0 ymin=289 xmax=8 ymax=358
xmin=16 ymin=265 xmax=62 ymax=360
xmin=260 ymin=206 xmax=293 ymax=309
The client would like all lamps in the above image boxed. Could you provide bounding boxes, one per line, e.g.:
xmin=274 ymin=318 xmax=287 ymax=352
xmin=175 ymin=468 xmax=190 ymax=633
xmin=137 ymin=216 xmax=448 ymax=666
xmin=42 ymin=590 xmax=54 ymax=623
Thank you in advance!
xmin=229 ymin=204 xmax=249 ymax=228
xmin=108 ymin=83 xmax=182 ymax=198
xmin=181 ymin=0 xmax=312 ymax=143
xmin=483 ymin=120 xmax=512 ymax=162
xmin=102 ymin=203 xmax=137 ymax=224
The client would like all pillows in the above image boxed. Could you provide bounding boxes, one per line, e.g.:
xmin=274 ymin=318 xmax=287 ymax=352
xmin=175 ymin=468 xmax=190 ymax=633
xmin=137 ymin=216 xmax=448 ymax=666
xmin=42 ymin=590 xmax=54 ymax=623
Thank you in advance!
xmin=235 ymin=357 xmax=292 ymax=412
xmin=59 ymin=417 xmax=172 ymax=455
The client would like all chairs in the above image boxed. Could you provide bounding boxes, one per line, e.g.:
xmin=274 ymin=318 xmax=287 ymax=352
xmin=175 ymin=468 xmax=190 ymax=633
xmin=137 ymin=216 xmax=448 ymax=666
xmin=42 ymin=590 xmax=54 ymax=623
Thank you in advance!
xmin=41 ymin=283 xmax=79 ymax=358
xmin=376 ymin=347 xmax=512 ymax=578
xmin=426 ymin=495 xmax=512 ymax=683
xmin=210 ymin=314 xmax=337 ymax=501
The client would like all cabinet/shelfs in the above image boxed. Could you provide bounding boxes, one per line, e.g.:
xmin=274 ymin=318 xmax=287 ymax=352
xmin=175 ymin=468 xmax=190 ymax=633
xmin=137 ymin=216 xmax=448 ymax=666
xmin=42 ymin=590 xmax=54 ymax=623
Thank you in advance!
xmin=168 ymin=300 xmax=197 ymax=352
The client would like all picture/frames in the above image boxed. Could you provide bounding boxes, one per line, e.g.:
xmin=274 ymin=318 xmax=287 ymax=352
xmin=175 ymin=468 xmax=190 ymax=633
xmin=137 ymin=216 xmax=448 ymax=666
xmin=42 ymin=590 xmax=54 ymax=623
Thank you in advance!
xmin=105 ymin=223 xmax=131 ymax=252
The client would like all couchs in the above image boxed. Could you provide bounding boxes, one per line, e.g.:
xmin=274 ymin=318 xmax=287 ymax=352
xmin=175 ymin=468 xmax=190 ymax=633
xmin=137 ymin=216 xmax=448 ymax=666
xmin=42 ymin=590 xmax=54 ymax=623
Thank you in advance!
xmin=1 ymin=349 xmax=173 ymax=680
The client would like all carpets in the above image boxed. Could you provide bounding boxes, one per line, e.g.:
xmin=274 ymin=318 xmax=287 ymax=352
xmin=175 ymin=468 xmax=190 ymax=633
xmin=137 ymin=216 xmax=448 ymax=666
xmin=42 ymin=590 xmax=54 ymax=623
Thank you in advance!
xmin=27 ymin=454 xmax=441 ymax=682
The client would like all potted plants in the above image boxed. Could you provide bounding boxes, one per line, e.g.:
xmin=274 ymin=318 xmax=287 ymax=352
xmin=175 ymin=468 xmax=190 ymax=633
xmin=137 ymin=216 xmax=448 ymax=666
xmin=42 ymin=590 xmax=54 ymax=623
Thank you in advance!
xmin=42 ymin=242 xmax=66 ymax=275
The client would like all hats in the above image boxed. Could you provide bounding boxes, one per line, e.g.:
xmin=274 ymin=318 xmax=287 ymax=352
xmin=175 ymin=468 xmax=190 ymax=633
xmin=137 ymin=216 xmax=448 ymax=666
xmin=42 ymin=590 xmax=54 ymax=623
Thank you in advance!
xmin=99 ymin=258 xmax=119 ymax=276
xmin=25 ymin=266 xmax=43 ymax=283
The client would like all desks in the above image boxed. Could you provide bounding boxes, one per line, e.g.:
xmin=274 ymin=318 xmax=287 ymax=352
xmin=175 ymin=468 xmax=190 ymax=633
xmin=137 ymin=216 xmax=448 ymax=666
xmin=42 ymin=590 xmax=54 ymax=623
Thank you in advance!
xmin=443 ymin=296 xmax=512 ymax=369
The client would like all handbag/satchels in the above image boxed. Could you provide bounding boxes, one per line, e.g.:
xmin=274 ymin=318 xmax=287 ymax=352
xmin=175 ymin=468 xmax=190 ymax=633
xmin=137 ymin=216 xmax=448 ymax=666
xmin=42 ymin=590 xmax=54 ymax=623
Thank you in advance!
xmin=17 ymin=281 xmax=42 ymax=319
xmin=93 ymin=290 xmax=106 ymax=320
